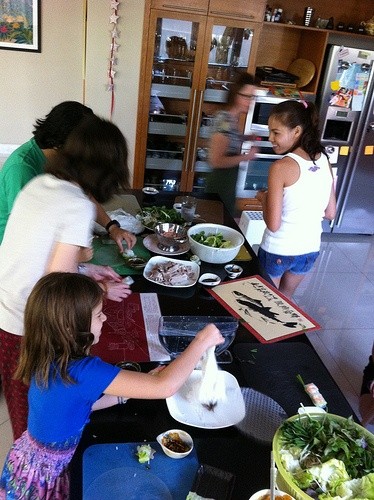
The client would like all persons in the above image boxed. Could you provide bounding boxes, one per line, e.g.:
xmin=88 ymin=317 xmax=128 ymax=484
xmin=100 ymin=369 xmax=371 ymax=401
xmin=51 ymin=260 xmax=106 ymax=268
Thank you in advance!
xmin=203 ymin=72 xmax=258 ymax=217
xmin=0 ymin=271 xmax=225 ymax=500
xmin=254 ymin=99 xmax=337 ymax=300
xmin=0 ymin=115 xmax=133 ymax=442
xmin=0 ymin=101 xmax=137 ymax=254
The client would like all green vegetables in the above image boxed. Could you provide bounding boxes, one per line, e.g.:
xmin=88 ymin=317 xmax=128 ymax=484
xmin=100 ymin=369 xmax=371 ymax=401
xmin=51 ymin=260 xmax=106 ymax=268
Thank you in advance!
xmin=190 ymin=231 xmax=235 ymax=248
xmin=138 ymin=205 xmax=185 ymax=225
xmin=279 ymin=403 xmax=374 ymax=480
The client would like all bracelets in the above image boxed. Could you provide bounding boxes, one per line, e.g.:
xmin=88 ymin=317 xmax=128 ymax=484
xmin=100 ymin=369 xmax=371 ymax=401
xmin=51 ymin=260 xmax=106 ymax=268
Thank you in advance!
xmin=118 ymin=396 xmax=126 ymax=404
xmin=97 ymin=280 xmax=108 ymax=297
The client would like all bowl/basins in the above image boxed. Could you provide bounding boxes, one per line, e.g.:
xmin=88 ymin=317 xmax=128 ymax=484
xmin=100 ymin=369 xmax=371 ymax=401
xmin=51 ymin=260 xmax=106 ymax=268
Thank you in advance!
xmin=156 ymin=429 xmax=194 ymax=460
xmin=127 ymin=256 xmax=147 ymax=270
xmin=295 ymin=402 xmax=328 ymax=415
xmin=187 ymin=223 xmax=245 ymax=265
xmin=113 ymin=361 xmax=141 ymax=373
xmin=248 ymin=489 xmax=296 ymax=500
xmin=155 ymin=223 xmax=188 ymax=252
xmin=225 ymin=264 xmax=244 ymax=279
xmin=157 ymin=314 xmax=238 ymax=359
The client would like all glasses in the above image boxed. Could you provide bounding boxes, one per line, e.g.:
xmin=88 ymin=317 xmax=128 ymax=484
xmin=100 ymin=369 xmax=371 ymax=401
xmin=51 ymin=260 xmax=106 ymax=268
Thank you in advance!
xmin=236 ymin=91 xmax=259 ymax=101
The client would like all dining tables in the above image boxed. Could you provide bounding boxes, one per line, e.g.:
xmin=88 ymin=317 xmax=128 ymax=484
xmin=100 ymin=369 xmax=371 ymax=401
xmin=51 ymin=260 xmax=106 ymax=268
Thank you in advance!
xmin=75 ymin=191 xmax=374 ymax=500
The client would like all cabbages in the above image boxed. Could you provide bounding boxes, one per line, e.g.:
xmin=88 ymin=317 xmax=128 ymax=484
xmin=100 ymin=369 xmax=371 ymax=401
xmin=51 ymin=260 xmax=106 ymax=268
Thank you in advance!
xmin=279 ymin=449 xmax=374 ymax=500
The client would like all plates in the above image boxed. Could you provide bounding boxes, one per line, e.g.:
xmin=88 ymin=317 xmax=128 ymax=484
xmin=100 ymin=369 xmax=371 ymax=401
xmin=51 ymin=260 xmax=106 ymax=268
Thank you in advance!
xmin=198 ymin=273 xmax=221 ymax=286
xmin=136 ymin=187 xmax=191 ymax=255
xmin=163 ymin=368 xmax=246 ymax=429
xmin=143 ymin=255 xmax=200 ymax=288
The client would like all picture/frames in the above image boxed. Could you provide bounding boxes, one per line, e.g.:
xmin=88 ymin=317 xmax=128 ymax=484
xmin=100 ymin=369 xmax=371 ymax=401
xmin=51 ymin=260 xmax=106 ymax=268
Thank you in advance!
xmin=0 ymin=0 xmax=41 ymax=54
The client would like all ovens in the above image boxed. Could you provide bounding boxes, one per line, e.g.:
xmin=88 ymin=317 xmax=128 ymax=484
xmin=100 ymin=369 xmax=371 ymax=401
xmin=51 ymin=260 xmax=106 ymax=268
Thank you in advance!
xmin=236 ymin=89 xmax=290 ymax=199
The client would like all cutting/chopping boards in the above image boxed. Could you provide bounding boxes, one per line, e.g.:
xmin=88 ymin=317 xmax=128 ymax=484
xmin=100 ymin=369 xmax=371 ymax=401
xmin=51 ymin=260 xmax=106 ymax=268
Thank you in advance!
xmin=84 ymin=236 xmax=150 ymax=276
xmin=288 ymin=58 xmax=315 ymax=88
xmin=81 ymin=442 xmax=200 ymax=500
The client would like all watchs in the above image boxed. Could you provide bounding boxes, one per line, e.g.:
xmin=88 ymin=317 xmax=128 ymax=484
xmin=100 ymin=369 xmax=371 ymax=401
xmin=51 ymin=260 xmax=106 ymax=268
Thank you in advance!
xmin=105 ymin=220 xmax=121 ymax=232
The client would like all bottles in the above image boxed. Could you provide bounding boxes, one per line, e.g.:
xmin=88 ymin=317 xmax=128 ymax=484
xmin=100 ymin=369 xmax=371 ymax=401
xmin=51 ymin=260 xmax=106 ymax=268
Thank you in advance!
xmin=153 ymin=28 xmax=240 ymax=92
xmin=265 ymin=5 xmax=334 ymax=30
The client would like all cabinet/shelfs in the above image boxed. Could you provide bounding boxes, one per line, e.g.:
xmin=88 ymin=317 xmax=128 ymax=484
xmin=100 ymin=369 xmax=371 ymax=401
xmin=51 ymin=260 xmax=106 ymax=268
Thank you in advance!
xmin=132 ymin=0 xmax=374 ymax=222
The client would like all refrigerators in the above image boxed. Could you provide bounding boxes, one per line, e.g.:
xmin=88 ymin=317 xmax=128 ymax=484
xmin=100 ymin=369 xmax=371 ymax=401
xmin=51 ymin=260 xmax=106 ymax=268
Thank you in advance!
xmin=317 ymin=44 xmax=374 ymax=235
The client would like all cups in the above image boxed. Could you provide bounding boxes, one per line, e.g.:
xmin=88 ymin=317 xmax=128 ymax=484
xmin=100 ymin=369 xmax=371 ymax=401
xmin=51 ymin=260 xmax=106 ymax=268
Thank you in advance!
xmin=181 ymin=196 xmax=197 ymax=226
